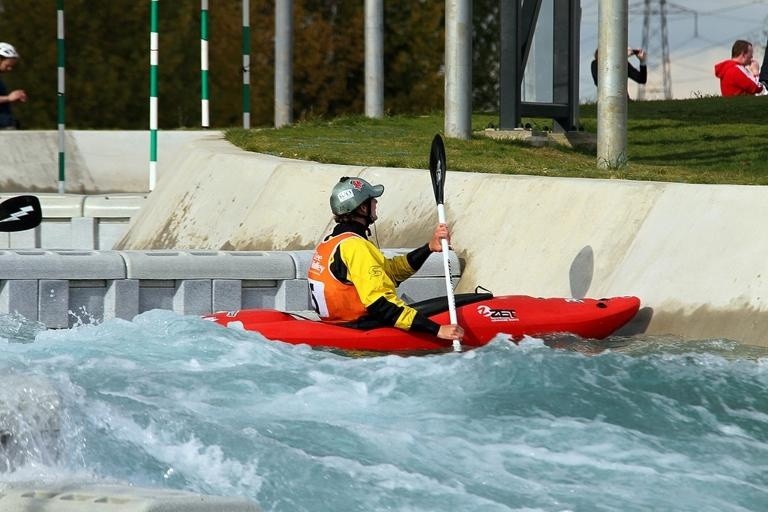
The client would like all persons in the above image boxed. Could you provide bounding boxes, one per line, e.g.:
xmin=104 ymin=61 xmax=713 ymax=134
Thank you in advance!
xmin=714 ymin=39 xmax=763 ymax=97
xmin=758 ymin=40 xmax=768 ymax=91
xmin=306 ymin=177 xmax=466 ymax=340
xmin=590 ymin=48 xmax=648 ymax=103
xmin=0 ymin=41 xmax=29 ymax=130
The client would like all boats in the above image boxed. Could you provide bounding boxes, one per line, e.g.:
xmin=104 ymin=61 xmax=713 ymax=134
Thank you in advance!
xmin=201 ymin=286 xmax=640 ymax=351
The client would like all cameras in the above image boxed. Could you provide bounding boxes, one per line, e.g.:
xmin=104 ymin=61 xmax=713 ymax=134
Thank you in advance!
xmin=633 ymin=49 xmax=639 ymax=54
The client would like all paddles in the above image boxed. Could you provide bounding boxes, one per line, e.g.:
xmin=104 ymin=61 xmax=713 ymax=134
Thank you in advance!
xmin=0 ymin=196 xmax=41 ymax=232
xmin=430 ymin=133 xmax=462 ymax=352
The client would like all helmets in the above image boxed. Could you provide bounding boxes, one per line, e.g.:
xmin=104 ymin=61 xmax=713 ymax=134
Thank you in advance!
xmin=330 ymin=176 xmax=384 ymax=216
xmin=1 ymin=42 xmax=19 ymax=59
